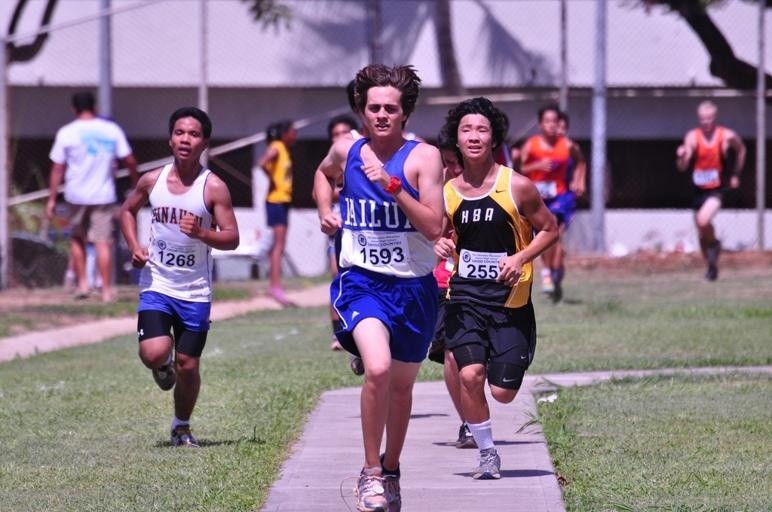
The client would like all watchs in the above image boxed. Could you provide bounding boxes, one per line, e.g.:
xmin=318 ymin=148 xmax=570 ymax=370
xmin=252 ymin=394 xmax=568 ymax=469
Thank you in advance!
xmin=384 ymin=175 xmax=401 ymax=193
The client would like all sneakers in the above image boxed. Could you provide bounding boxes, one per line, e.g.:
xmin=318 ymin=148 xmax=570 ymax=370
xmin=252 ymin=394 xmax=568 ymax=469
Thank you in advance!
xmin=350 ymin=354 xmax=365 ymax=375
xmin=378 ymin=453 xmax=401 ymax=512
xmin=703 ymin=239 xmax=720 ymax=282
xmin=265 ymin=286 xmax=295 ymax=306
xmin=473 ymin=448 xmax=501 ymax=479
xmin=152 ymin=334 xmax=176 ymax=391
xmin=540 ymin=265 xmax=564 ymax=305
xmin=353 ymin=465 xmax=389 ymax=512
xmin=65 ymin=273 xmax=118 ymax=306
xmin=456 ymin=422 xmax=478 ymax=448
xmin=332 ymin=332 xmax=345 ymax=350
xmin=170 ymin=418 xmax=199 ymax=448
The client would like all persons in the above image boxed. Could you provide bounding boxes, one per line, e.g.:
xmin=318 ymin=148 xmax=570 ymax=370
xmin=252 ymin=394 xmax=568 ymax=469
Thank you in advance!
xmin=45 ymin=90 xmax=138 ymax=302
xmin=115 ymin=106 xmax=239 ymax=447
xmin=313 ymin=105 xmax=588 ymax=374
xmin=258 ymin=118 xmax=297 ymax=308
xmin=673 ymin=100 xmax=746 ymax=280
xmin=315 ymin=65 xmax=444 ymax=511
xmin=434 ymin=96 xmax=561 ymax=480
xmin=429 ymin=123 xmax=490 ymax=448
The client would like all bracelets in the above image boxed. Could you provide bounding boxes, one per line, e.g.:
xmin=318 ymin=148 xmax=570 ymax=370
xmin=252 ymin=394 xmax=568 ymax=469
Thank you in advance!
xmin=730 ymin=169 xmax=742 ymax=176
